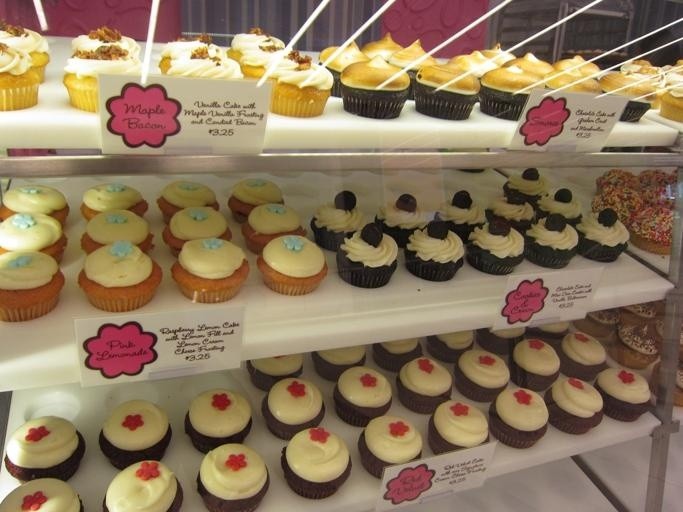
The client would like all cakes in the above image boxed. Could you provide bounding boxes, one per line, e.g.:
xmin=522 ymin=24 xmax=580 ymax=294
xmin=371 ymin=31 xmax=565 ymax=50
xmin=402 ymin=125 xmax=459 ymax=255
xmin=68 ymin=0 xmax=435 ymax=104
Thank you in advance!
xmin=573 ymin=310 xmax=621 ymax=335
xmin=372 ymin=338 xmax=423 ymax=373
xmin=80 ymin=182 xmax=149 ymax=222
xmin=62 ymin=46 xmax=142 ymax=113
xmin=523 ymin=213 xmax=579 ymax=269
xmin=171 ymin=237 xmax=250 ymax=303
xmin=543 ymin=377 xmax=604 ymax=435
xmin=476 ymin=327 xmax=525 ymax=354
xmin=628 ymin=65 xmax=665 ymax=110
xmin=480 ymin=42 xmax=516 ymax=68
xmin=267 ymin=52 xmax=334 ymax=118
xmin=156 ymin=179 xmax=220 ymax=225
xmin=340 ymin=54 xmax=411 ymax=119
xmin=544 ymin=65 xmax=601 ymax=94
xmin=608 ymin=323 xmax=658 ymax=369
xmin=501 ymin=52 xmax=554 ymax=77
xmin=166 ymin=46 xmax=244 ymax=82
xmin=228 ymin=26 xmax=285 ymax=62
xmin=428 ymin=400 xmax=490 ymax=456
xmin=103 ymin=460 xmax=184 ymax=512
xmin=374 ymin=194 xmax=436 ymax=248
xmin=162 ymin=206 xmax=232 ymax=259
xmin=99 ymin=399 xmax=172 ymax=471
xmin=427 ymin=330 xmax=474 ymax=364
xmin=256 ymin=234 xmax=328 ymax=296
xmin=336 ymin=223 xmax=398 ymax=288
xmin=599 ymin=73 xmax=663 ymax=123
xmin=489 ymin=387 xmax=549 ymax=449
xmin=455 ymin=349 xmax=510 ymax=402
xmin=281 ymin=427 xmax=353 ymax=499
xmin=404 ymin=220 xmax=465 ymax=281
xmin=158 ymin=32 xmax=227 ymax=75
xmin=480 ymin=65 xmax=545 ymax=123
xmin=239 ymin=41 xmax=296 ymax=81
xmin=503 ymin=167 xmax=549 ymax=211
xmin=261 ymin=376 xmax=326 ymax=441
xmin=434 ymin=190 xmax=486 ymax=244
xmin=396 ymin=355 xmax=455 ymax=414
xmin=558 ymin=332 xmax=607 ymax=380
xmin=414 ymin=56 xmax=480 ymax=121
xmin=246 ymin=352 xmax=304 ymax=392
xmin=0 ymin=211 xmax=67 ymax=266
xmin=484 ymin=192 xmax=538 ymax=235
xmin=228 ymin=179 xmax=285 ymax=224
xmin=593 ymin=367 xmax=652 ymax=422
xmin=81 ymin=209 xmax=154 ymax=258
xmin=358 ymin=415 xmax=423 ymax=480
xmin=509 ymin=338 xmax=561 ymax=392
xmin=361 ymin=31 xmax=403 ymax=63
xmin=241 ymin=203 xmax=307 ymax=255
xmin=387 ymin=38 xmax=437 ymax=101
xmin=649 ymin=361 xmax=683 ymax=407
xmin=620 ymin=59 xmax=652 ymax=75
xmin=333 ymin=366 xmax=393 ymax=428
xmin=0 ymin=184 xmax=70 ymax=229
xmin=185 ymin=388 xmax=253 ymax=455
xmin=196 ymin=443 xmax=270 ymax=511
xmin=0 ymin=20 xmax=49 ymax=84
xmin=0 ymin=250 xmax=65 ymax=323
xmin=616 ymin=302 xmax=656 ymax=325
xmin=552 ymin=54 xmax=601 ymax=80
xmin=576 ymin=168 xmax=676 ymax=263
xmin=4 ymin=415 xmax=86 ymax=485
xmin=536 ymin=188 xmax=583 ymax=227
xmin=317 ymin=41 xmax=370 ymax=98
xmin=448 ymin=49 xmax=501 ymax=101
xmin=466 ymin=215 xmax=527 ymax=275
xmin=659 ymin=85 xmax=683 ymax=123
xmin=71 ymin=26 xmax=142 ymax=56
xmin=0 ymin=41 xmax=39 ymax=111
xmin=310 ymin=190 xmax=367 ymax=252
xmin=0 ymin=477 xmax=84 ymax=512
xmin=311 ymin=344 xmax=366 ymax=382
xmin=78 ymin=240 xmax=163 ymax=312
xmin=651 ymin=315 xmax=683 ymax=358
xmin=526 ymin=321 xmax=570 ymax=343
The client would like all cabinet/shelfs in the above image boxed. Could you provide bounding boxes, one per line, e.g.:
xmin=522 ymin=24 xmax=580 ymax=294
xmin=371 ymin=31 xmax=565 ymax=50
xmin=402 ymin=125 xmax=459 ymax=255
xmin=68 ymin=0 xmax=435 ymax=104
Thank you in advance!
xmin=2 ymin=37 xmax=683 ymax=512
